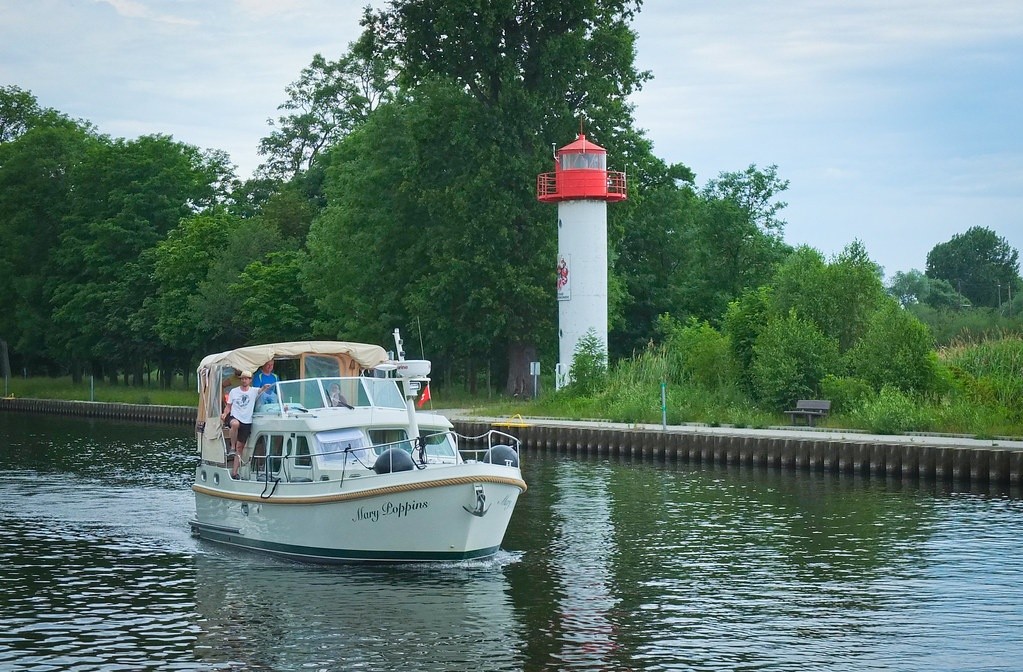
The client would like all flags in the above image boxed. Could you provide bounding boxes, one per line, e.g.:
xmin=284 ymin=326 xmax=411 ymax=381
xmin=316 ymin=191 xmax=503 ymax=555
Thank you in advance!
xmin=418 ymin=385 xmax=431 ymax=407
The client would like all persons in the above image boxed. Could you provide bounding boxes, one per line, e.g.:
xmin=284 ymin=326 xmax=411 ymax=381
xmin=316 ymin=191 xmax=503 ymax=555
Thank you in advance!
xmin=321 ymin=383 xmax=348 ymax=407
xmin=253 ymin=360 xmax=284 ymax=412
xmin=222 ymin=379 xmax=234 ymax=410
xmin=220 ymin=371 xmax=271 ymax=480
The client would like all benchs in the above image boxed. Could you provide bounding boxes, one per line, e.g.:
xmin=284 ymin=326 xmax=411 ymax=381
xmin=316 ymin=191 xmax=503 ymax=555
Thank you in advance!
xmin=784 ymin=400 xmax=831 ymax=426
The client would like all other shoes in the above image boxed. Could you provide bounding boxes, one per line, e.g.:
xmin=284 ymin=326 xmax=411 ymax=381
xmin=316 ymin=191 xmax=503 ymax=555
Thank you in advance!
xmin=228 ymin=449 xmax=236 ymax=457
xmin=230 ymin=474 xmax=243 ymax=480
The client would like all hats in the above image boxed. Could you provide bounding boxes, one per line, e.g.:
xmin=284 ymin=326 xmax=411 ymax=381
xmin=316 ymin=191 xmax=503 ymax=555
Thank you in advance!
xmin=237 ymin=371 xmax=252 ymax=379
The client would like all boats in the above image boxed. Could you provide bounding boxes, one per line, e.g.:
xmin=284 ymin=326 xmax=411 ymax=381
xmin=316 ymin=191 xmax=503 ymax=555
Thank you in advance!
xmin=188 ymin=327 xmax=528 ymax=566
